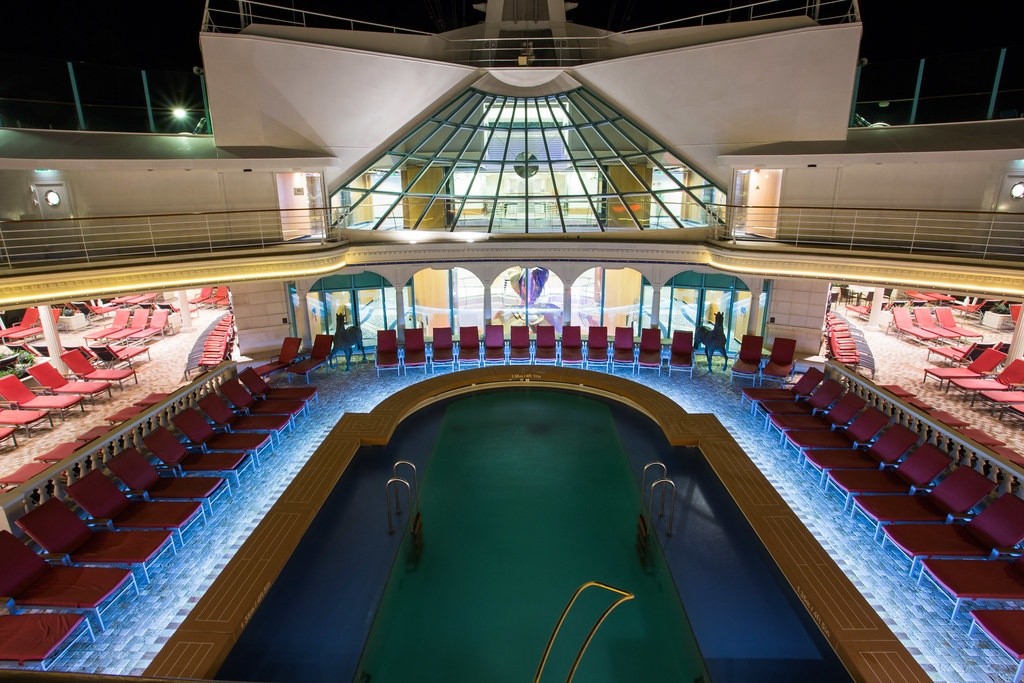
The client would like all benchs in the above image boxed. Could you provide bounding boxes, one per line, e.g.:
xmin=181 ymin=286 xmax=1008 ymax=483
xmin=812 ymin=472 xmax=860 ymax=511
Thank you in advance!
xmin=460 ymin=202 xmax=600 ymax=226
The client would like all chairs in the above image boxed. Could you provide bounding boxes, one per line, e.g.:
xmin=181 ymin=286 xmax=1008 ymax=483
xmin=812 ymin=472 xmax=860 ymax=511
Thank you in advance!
xmin=730 ymin=287 xmax=1024 ymax=683
xmin=0 ymin=286 xmax=335 ymax=671
xmin=375 ymin=325 xmax=695 ymax=381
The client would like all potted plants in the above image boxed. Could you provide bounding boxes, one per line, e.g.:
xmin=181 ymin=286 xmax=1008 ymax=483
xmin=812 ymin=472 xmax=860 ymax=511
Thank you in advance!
xmin=982 ymin=302 xmax=1014 ymax=330
xmin=58 ymin=309 xmax=86 ymax=329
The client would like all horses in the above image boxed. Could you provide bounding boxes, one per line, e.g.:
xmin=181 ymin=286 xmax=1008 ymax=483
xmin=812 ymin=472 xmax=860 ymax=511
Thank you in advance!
xmin=329 ymin=314 xmax=367 ymax=371
xmin=692 ymin=312 xmax=729 ymax=372
